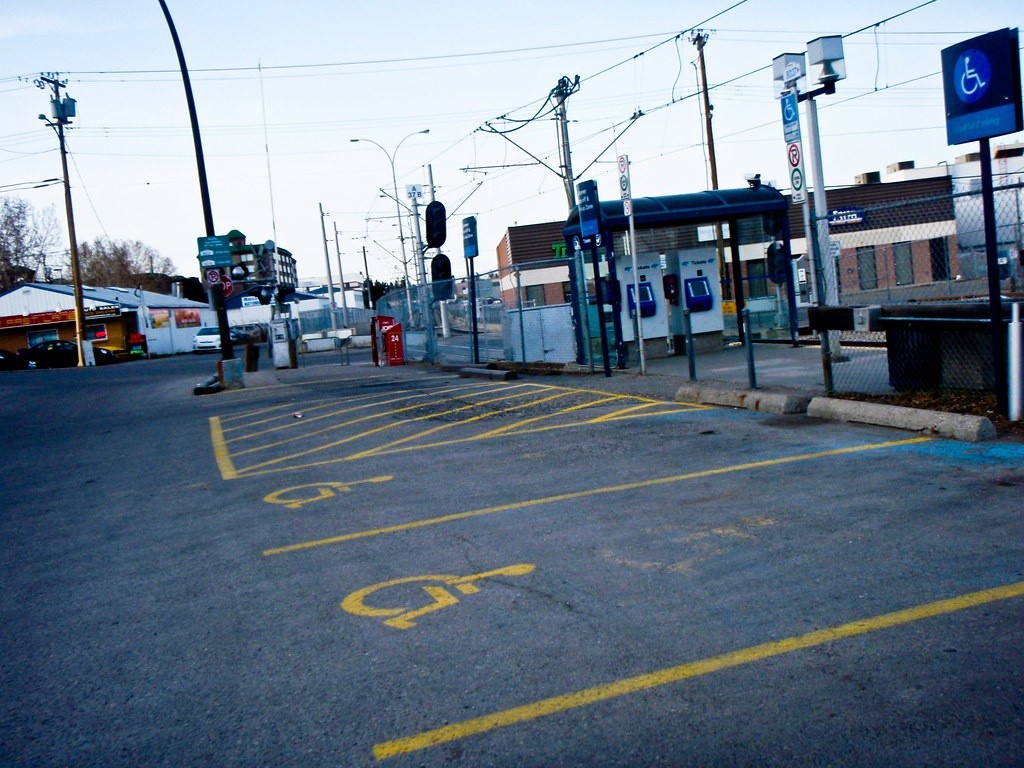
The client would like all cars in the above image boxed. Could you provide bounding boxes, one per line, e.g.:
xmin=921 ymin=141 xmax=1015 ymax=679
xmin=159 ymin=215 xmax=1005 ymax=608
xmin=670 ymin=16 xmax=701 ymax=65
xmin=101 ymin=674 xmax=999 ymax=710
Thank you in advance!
xmin=229 ymin=323 xmax=271 ymax=344
xmin=16 ymin=339 xmax=115 ymax=370
xmin=193 ymin=325 xmax=222 ymax=354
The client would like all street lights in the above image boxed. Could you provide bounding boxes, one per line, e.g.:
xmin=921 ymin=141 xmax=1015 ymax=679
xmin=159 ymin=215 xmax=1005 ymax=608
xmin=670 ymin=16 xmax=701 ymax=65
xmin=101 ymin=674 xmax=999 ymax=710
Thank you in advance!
xmin=350 ymin=130 xmax=431 ymax=329
xmin=38 ymin=113 xmax=87 ymax=366
